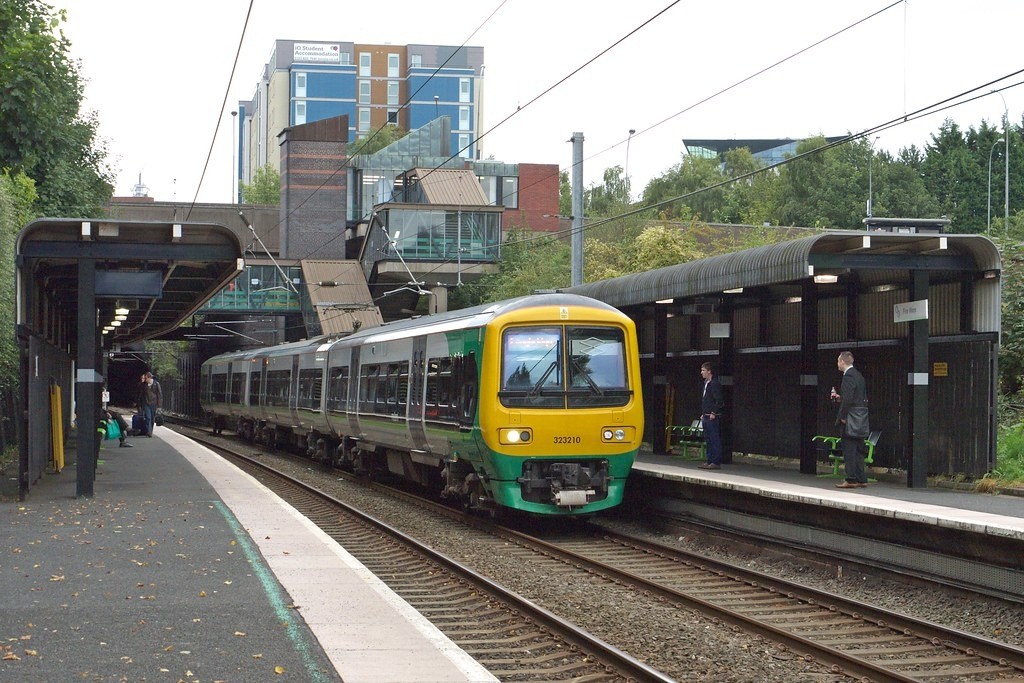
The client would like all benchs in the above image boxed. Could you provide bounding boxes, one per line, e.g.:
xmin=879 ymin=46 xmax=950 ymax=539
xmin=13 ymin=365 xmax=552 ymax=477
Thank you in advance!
xmin=71 ymin=418 xmax=109 ymax=467
xmin=812 ymin=430 xmax=882 ymax=484
xmin=665 ymin=418 xmax=711 ymax=460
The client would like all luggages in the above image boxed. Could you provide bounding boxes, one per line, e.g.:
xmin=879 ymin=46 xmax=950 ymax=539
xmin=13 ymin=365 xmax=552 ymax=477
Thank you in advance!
xmin=131 ymin=415 xmax=149 ymax=436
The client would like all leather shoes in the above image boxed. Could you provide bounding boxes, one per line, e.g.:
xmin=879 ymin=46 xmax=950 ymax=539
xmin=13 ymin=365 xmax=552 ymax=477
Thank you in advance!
xmin=856 ymin=482 xmax=868 ymax=487
xmin=835 ymin=481 xmax=858 ymax=488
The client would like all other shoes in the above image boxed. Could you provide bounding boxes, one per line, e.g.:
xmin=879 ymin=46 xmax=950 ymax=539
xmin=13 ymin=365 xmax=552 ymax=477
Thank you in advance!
xmin=697 ymin=462 xmax=710 ymax=468
xmin=704 ymin=463 xmax=721 ymax=469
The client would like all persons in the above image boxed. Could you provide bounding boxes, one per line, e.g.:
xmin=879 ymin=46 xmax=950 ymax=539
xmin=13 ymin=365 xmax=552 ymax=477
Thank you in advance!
xmin=697 ymin=362 xmax=725 ymax=470
xmin=830 ymin=351 xmax=870 ymax=489
xmin=97 ymin=371 xmax=164 ymax=448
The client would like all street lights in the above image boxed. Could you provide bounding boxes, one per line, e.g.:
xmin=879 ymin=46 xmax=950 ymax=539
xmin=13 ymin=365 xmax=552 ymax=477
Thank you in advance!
xmin=987 ymin=138 xmax=1006 ymax=235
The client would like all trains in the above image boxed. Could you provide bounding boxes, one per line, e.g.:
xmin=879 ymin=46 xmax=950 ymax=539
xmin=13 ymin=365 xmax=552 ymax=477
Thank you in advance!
xmin=200 ymin=292 xmax=646 ymax=534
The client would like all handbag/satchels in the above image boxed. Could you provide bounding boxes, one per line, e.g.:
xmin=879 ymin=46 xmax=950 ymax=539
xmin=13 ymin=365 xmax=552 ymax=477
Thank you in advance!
xmin=106 ymin=418 xmax=122 ymax=440
xmin=156 ymin=408 xmax=164 ymax=426
xmin=844 ymin=406 xmax=870 ymax=440
